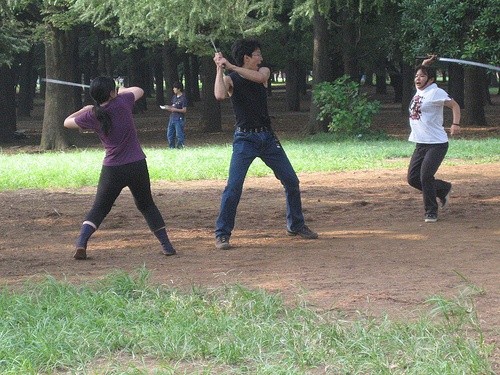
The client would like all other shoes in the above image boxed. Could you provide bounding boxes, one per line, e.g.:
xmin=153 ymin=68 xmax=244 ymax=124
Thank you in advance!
xmin=74 ymin=248 xmax=86 ymax=259
xmin=163 ymin=249 xmax=176 ymax=255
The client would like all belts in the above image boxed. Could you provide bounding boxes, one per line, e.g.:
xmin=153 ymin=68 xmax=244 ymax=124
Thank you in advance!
xmin=237 ymin=126 xmax=270 ymax=133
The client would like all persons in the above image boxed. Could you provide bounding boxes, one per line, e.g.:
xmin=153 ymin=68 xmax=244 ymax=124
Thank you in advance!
xmin=407 ymin=55 xmax=460 ymax=222
xmin=167 ymin=82 xmax=187 ymax=150
xmin=63 ymin=77 xmax=176 ymax=258
xmin=213 ymin=39 xmax=318 ymax=249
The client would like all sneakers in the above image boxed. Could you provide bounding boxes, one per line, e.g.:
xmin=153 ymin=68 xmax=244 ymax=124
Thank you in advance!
xmin=287 ymin=225 xmax=318 ymax=239
xmin=216 ymin=235 xmax=230 ymax=249
xmin=424 ymin=213 xmax=437 ymax=223
xmin=440 ymin=192 xmax=450 ymax=209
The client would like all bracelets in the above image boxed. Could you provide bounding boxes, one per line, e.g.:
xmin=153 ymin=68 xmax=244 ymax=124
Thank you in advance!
xmin=453 ymin=123 xmax=460 ymax=126
